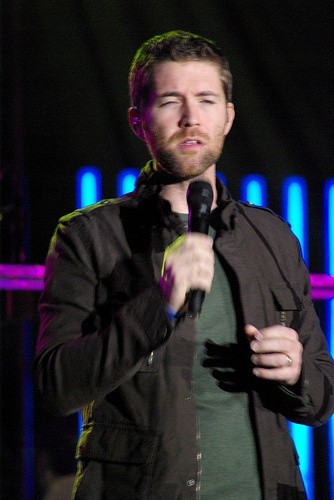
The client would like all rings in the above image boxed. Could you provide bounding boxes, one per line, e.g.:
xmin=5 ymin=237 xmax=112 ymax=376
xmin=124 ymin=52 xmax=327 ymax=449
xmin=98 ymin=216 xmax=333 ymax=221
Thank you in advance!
xmin=284 ymin=353 xmax=293 ymax=367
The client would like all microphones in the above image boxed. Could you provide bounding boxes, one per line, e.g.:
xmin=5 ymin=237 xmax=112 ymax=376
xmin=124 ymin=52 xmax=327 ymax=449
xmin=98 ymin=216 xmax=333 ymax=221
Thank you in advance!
xmin=185 ymin=179 xmax=213 ymax=320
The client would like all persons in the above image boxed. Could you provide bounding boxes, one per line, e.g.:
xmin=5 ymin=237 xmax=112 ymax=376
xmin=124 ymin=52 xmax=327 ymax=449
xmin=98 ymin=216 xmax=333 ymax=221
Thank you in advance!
xmin=35 ymin=30 xmax=334 ymax=500
